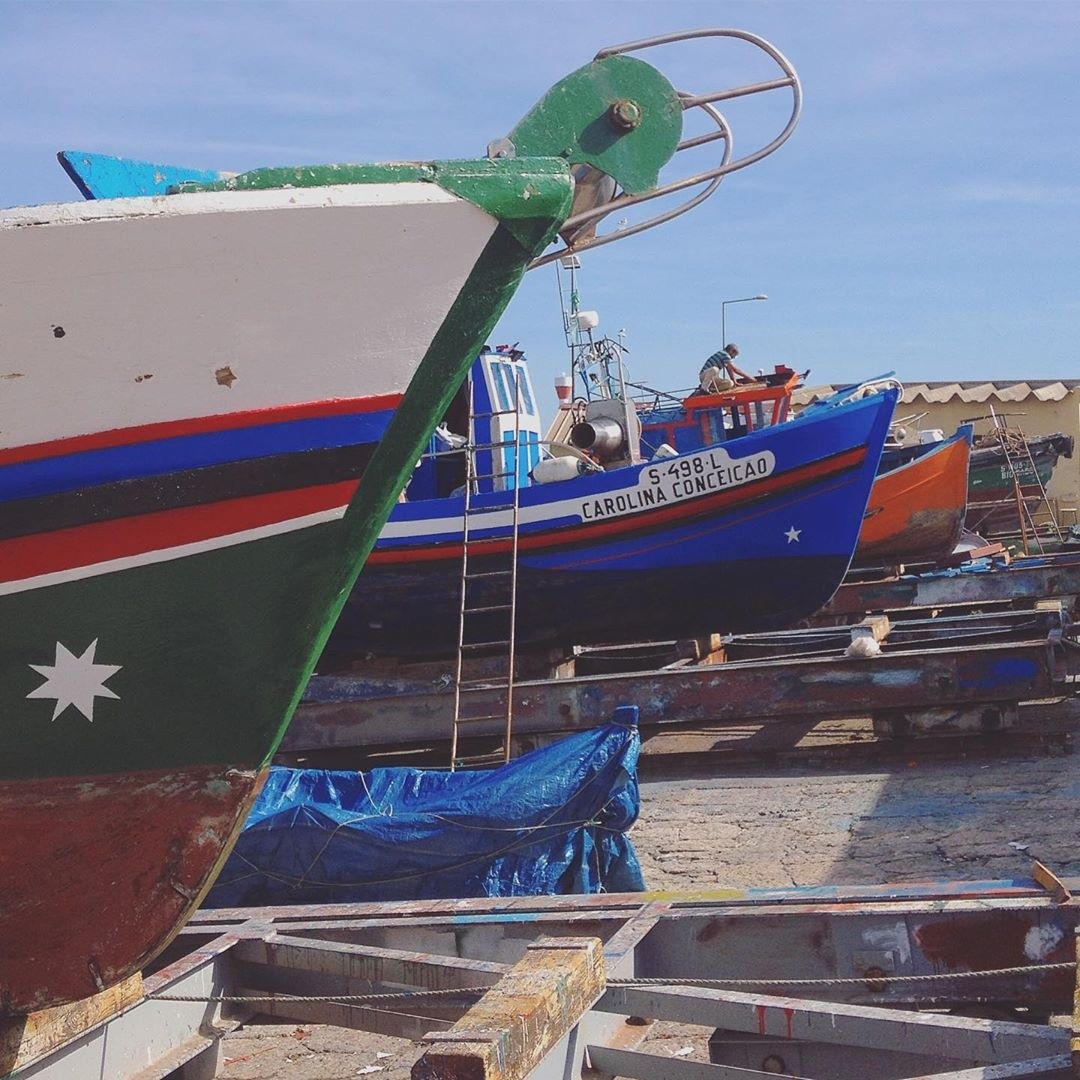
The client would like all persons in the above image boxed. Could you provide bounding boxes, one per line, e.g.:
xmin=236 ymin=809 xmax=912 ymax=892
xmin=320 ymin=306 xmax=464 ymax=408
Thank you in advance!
xmin=698 ymin=343 xmax=758 ymax=395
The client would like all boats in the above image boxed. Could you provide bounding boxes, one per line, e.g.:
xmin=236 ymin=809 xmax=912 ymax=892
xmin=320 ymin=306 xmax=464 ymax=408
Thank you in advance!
xmin=320 ymin=346 xmax=904 ymax=645
xmin=0 ymin=19 xmax=806 ymax=1016
xmin=855 ymin=417 xmax=976 ymax=564
xmin=966 ymin=434 xmax=1072 ymax=539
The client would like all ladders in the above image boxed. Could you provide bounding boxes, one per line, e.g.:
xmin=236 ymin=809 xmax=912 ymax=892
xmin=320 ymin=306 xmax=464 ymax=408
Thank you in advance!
xmin=998 ymin=424 xmax=1064 ymax=555
xmin=450 ymin=373 xmax=519 ymax=773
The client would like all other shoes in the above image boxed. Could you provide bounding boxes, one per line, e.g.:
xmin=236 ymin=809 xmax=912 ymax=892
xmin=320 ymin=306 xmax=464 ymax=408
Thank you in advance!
xmin=698 ymin=387 xmax=716 ymax=395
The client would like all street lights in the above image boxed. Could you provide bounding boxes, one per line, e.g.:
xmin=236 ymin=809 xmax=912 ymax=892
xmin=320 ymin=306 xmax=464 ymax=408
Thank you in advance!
xmin=722 ymin=296 xmax=767 ymax=378
